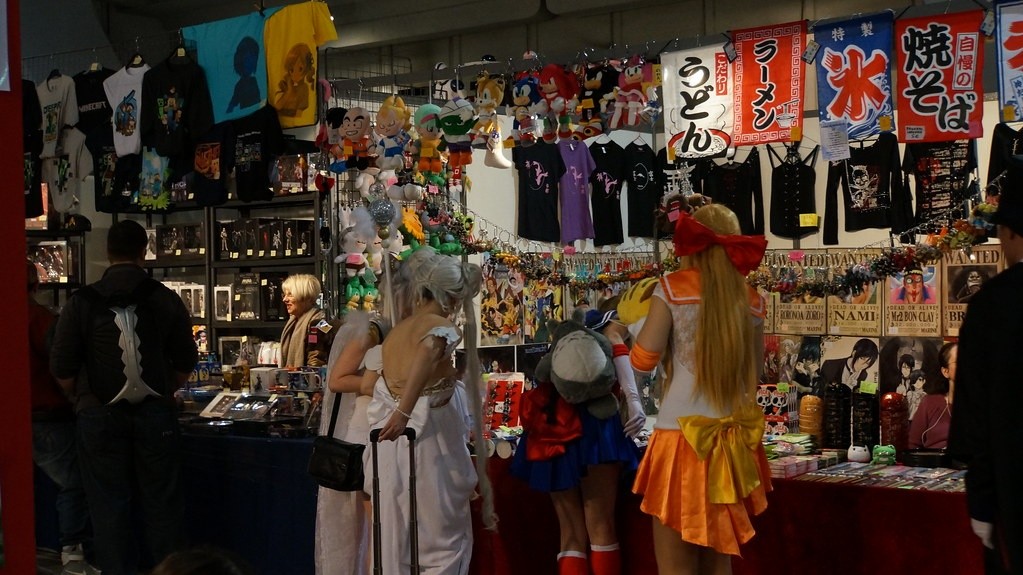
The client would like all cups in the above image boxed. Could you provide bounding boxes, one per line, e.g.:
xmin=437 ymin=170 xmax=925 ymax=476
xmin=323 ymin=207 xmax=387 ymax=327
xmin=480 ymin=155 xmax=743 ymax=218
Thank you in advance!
xmin=274 ymin=368 xmax=289 ymax=388
xmin=288 ymin=371 xmax=321 ymax=393
xmin=278 ymin=395 xmax=311 ymax=418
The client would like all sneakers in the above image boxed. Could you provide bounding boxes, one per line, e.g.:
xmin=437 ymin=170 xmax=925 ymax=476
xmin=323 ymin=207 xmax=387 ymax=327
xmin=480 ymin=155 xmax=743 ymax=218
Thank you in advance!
xmin=60 ymin=543 xmax=102 ymax=575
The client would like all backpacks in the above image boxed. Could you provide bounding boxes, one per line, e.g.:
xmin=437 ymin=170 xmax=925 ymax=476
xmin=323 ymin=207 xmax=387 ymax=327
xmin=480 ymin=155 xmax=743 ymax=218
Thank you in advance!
xmin=535 ymin=307 xmax=622 ymax=418
xmin=80 ymin=277 xmax=176 ymax=408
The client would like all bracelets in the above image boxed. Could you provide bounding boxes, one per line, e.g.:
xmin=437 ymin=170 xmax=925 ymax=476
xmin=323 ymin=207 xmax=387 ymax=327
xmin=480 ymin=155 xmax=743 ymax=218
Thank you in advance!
xmin=396 ymin=408 xmax=412 ymax=419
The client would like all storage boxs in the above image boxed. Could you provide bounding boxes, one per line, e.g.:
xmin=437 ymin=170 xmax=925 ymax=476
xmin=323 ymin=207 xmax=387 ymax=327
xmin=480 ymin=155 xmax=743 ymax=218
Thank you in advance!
xmin=145 ymin=221 xmax=310 ymax=392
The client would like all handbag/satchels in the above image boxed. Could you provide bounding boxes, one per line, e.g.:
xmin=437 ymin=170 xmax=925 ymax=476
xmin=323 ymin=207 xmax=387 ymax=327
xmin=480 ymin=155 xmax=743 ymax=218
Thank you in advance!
xmin=520 ymin=376 xmax=585 ymax=462
xmin=305 ymin=435 xmax=366 ymax=492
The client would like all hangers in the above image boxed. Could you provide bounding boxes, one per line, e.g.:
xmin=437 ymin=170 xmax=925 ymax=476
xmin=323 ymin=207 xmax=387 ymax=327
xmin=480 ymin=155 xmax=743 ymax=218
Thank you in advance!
xmin=125 ymin=36 xmax=146 ymax=69
xmin=46 ymin=54 xmax=62 ymax=83
xmin=632 ymin=132 xmax=646 ymax=143
xmin=82 ymin=48 xmax=105 ymax=75
xmin=594 ymin=133 xmax=611 ymax=142
xmin=167 ymin=28 xmax=192 ymax=64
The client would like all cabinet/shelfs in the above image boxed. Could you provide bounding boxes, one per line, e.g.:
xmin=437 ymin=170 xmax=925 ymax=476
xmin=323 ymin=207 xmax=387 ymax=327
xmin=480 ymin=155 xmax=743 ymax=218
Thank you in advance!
xmin=210 ymin=194 xmax=321 ymax=365
xmin=111 ymin=191 xmax=210 ymax=365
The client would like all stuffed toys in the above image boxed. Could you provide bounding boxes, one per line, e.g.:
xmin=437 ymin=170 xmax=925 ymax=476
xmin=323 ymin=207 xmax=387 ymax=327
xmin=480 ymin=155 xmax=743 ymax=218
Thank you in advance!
xmin=503 ymin=56 xmax=663 ymax=148
xmin=335 ymin=203 xmax=466 ymax=317
xmin=325 ymin=72 xmax=506 ymax=202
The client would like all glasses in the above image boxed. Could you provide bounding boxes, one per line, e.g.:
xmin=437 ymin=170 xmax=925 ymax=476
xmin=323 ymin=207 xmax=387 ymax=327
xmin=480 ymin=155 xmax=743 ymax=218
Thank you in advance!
xmin=281 ymin=291 xmax=294 ymax=300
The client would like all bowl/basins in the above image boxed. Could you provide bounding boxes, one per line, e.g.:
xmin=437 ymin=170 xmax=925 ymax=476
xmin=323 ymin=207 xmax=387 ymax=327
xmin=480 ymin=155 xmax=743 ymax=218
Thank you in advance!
xmin=799 ymin=383 xmax=947 ymax=467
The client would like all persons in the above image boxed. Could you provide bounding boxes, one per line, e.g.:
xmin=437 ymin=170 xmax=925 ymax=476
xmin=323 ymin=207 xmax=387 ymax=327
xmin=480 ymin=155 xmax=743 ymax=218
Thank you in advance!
xmin=510 ymin=295 xmax=647 ymax=575
xmin=28 ymin=259 xmax=103 ymax=575
xmin=628 ymin=205 xmax=773 ymax=575
xmin=170 ymin=228 xmax=177 ymax=249
xmin=197 ymin=333 xmax=207 ymax=350
xmin=51 ymin=219 xmax=197 ymax=575
xmin=280 ymin=275 xmax=331 ymax=368
xmin=947 ymin=154 xmax=1023 ymax=574
xmin=314 ymin=247 xmax=498 ymax=575
xmin=153 ymin=549 xmax=241 ymax=575
xmin=221 ymin=227 xmax=306 ymax=250
xmin=909 ymin=343 xmax=957 ymax=448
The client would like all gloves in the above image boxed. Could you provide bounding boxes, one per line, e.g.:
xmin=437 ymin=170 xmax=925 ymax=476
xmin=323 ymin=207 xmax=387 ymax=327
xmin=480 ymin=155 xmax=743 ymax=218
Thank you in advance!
xmin=608 ymin=343 xmax=646 ymax=441
xmin=628 ymin=350 xmax=655 ymax=396
